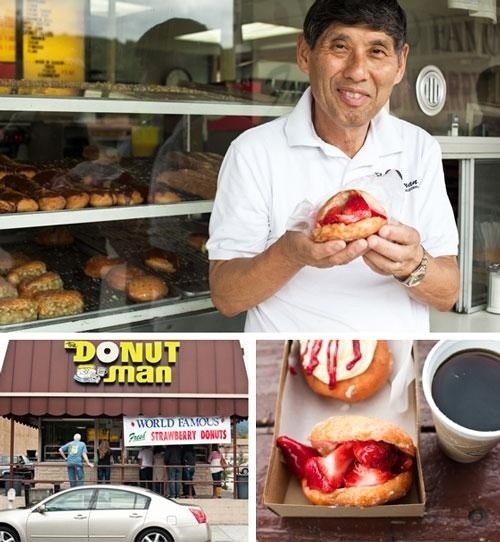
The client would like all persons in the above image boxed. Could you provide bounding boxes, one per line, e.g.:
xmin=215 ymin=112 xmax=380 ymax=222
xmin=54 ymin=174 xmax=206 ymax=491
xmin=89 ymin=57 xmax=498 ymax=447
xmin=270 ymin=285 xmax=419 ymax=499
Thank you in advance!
xmin=59 ymin=433 xmax=228 ymax=498
xmin=134 ymin=17 xmax=226 ymax=246
xmin=471 ymin=67 xmax=500 ymax=134
xmin=204 ymin=1 xmax=463 ymax=333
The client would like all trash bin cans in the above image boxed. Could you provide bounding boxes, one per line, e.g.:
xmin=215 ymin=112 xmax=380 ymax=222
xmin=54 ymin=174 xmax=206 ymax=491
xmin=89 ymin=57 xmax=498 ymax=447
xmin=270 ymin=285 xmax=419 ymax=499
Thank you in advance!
xmin=237 ymin=474 xmax=248 ymax=499
xmin=4 ymin=472 xmax=24 ymax=496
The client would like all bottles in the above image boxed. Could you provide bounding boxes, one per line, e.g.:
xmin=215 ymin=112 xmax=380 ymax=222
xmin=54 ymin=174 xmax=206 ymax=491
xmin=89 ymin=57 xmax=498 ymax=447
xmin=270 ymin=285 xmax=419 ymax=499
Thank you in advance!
xmin=452 ymin=117 xmax=460 ymax=136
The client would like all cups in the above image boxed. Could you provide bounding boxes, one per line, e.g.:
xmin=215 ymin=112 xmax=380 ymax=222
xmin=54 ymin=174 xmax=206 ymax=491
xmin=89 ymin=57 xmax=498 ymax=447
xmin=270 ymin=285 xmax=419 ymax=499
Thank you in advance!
xmin=422 ymin=338 xmax=500 ymax=465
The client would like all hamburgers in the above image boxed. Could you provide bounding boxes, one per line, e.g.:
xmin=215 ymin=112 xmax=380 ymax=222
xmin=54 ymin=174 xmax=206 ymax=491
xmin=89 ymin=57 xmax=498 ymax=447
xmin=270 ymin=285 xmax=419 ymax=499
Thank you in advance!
xmin=275 ymin=414 xmax=415 ymax=510
xmin=298 ymin=338 xmax=393 ymax=405
xmin=1 ymin=219 xmax=85 ymax=331
xmin=311 ymin=188 xmax=391 ymax=244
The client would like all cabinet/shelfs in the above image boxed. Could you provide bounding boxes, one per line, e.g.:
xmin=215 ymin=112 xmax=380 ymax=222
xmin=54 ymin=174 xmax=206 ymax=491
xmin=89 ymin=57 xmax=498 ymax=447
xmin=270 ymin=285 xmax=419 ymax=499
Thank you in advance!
xmin=395 ymin=13 xmax=500 ymax=128
xmin=194 ymin=446 xmax=208 ymax=464
xmin=0 ymin=82 xmax=298 ymax=332
xmin=43 ymin=444 xmax=95 ymax=462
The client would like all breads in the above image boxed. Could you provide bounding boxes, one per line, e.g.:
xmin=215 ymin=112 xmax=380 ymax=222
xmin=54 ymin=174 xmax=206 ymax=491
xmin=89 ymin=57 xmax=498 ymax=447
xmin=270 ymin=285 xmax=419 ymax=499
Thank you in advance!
xmin=0 ymin=126 xmax=226 ymax=214
xmin=85 ymin=231 xmax=211 ymax=303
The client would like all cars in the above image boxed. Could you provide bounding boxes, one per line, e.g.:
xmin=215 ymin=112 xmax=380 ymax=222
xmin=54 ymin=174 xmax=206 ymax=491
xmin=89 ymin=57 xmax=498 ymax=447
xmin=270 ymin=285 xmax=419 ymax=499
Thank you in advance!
xmin=238 ymin=462 xmax=248 ymax=474
xmin=0 ymin=483 xmax=212 ymax=541
xmin=0 ymin=455 xmax=35 ymax=481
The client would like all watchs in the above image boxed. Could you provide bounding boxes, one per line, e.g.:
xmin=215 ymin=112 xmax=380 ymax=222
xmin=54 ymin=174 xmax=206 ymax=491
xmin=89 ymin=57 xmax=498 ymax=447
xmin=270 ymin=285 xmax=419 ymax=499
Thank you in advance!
xmin=392 ymin=245 xmax=431 ymax=289
